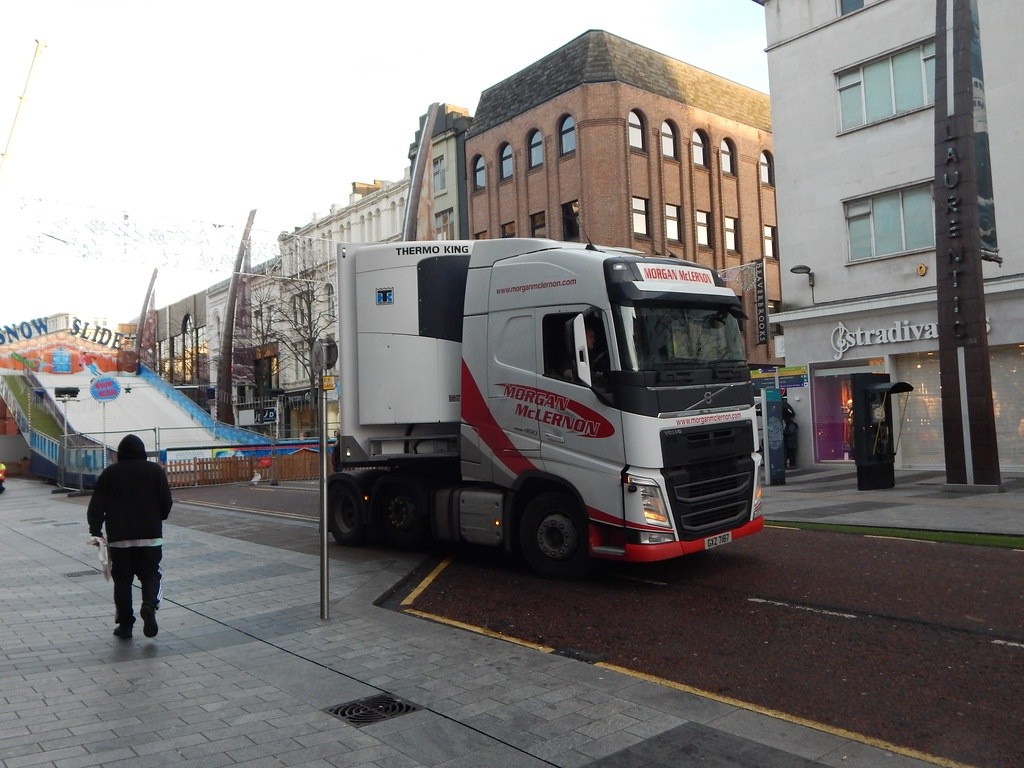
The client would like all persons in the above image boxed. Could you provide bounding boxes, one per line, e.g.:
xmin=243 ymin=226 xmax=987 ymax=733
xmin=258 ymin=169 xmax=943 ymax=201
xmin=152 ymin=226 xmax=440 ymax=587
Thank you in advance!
xmin=784 ymin=417 xmax=797 ymax=467
xmin=87 ymin=434 xmax=173 ymax=638
xmin=0 ymin=464 xmax=7 ymax=494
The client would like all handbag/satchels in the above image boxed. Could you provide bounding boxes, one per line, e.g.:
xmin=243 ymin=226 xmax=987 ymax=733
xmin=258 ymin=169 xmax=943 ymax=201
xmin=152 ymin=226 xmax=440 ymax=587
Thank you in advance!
xmin=86 ymin=536 xmax=112 ymax=584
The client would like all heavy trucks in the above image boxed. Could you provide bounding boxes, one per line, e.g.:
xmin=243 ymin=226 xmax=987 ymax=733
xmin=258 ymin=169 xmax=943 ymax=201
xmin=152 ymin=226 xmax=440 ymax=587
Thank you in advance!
xmin=328 ymin=237 xmax=763 ymax=580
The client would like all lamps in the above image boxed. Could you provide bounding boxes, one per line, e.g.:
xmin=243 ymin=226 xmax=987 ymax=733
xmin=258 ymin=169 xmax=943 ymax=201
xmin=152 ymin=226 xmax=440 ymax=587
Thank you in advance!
xmin=790 ymin=265 xmax=815 ymax=287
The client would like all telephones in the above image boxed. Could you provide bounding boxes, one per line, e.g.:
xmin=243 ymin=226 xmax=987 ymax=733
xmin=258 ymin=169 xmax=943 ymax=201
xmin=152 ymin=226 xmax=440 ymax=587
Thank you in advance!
xmin=871 ymin=402 xmax=886 ymax=424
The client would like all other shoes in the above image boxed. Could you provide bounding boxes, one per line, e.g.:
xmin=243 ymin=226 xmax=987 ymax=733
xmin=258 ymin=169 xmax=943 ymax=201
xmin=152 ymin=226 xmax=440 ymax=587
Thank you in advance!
xmin=113 ymin=626 xmax=132 ymax=639
xmin=140 ymin=605 xmax=159 ymax=638
